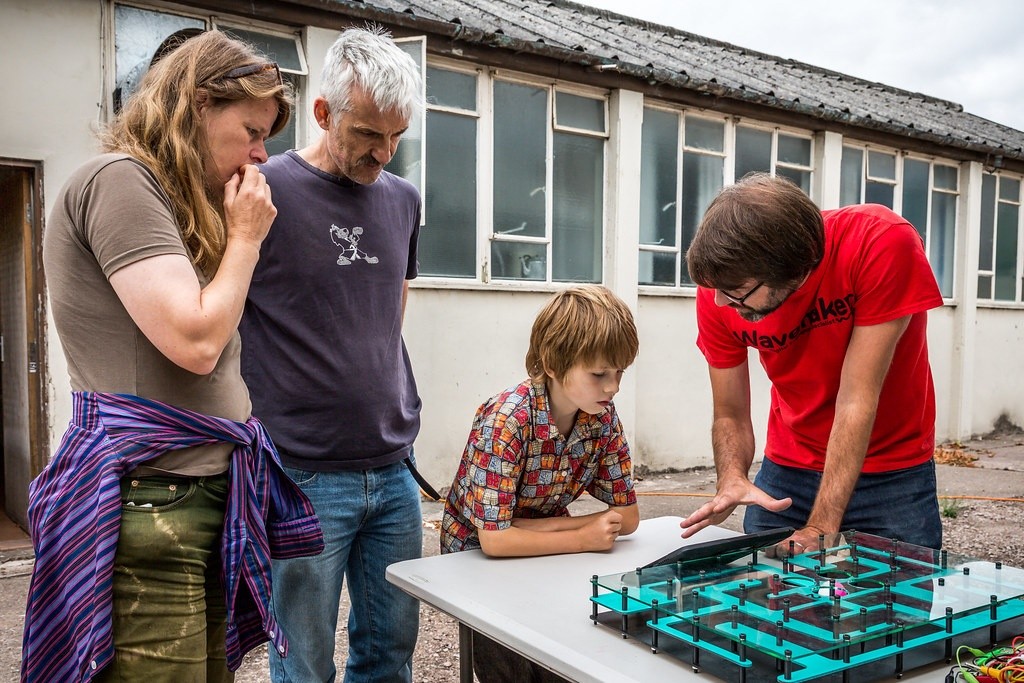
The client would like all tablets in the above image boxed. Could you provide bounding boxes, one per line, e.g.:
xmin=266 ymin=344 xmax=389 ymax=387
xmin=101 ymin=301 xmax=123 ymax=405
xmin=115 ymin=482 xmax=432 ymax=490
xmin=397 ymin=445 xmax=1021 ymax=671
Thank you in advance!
xmin=620 ymin=526 xmax=796 ymax=582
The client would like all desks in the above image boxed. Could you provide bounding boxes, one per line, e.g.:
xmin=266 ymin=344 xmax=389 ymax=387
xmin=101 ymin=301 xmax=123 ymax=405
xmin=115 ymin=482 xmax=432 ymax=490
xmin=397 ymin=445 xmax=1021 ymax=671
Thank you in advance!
xmin=386 ymin=513 xmax=1024 ymax=683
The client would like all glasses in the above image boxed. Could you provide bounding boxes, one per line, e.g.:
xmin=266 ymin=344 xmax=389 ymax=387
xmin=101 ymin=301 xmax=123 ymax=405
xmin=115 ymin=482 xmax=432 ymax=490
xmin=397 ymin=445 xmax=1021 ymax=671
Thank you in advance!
xmin=720 ymin=282 xmax=764 ymax=307
xmin=217 ymin=62 xmax=284 ymax=96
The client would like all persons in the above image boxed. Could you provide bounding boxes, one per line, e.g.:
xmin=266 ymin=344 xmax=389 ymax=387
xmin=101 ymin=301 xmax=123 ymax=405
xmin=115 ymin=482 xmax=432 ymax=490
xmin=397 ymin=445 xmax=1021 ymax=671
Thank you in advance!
xmin=27 ymin=24 xmax=301 ymax=683
xmin=677 ymin=172 xmax=945 ymax=573
xmin=438 ymin=286 xmax=639 ymax=682
xmin=224 ymin=19 xmax=425 ymax=683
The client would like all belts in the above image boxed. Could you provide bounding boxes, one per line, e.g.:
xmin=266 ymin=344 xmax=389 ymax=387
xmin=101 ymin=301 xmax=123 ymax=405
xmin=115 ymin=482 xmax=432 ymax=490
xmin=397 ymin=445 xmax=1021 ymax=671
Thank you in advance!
xmin=404 ymin=457 xmax=441 ymax=501
